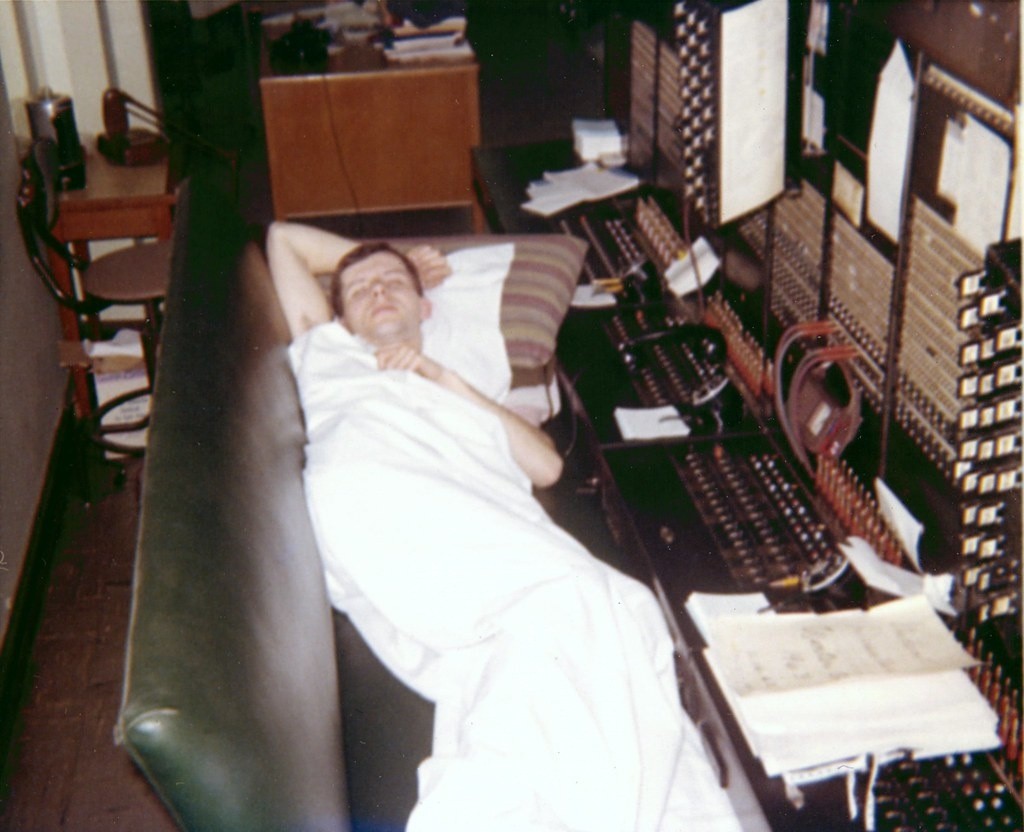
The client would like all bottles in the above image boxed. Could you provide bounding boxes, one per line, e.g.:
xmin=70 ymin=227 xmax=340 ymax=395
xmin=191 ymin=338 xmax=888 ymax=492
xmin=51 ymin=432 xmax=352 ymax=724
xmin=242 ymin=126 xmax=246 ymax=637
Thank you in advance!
xmin=23 ymin=87 xmax=87 ymax=189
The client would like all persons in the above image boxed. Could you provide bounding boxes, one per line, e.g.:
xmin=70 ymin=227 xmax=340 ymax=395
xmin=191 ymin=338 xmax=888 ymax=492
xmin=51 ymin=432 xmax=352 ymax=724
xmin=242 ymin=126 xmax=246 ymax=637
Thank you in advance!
xmin=266 ymin=218 xmax=563 ymax=487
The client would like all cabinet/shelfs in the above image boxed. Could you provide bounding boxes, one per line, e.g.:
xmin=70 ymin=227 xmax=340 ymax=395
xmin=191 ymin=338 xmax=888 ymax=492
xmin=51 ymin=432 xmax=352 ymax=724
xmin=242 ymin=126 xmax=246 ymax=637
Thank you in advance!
xmin=260 ymin=12 xmax=486 ymax=236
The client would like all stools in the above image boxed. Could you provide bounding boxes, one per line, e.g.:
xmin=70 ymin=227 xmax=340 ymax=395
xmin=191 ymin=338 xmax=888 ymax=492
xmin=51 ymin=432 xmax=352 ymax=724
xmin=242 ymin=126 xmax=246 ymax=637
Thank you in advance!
xmin=83 ymin=240 xmax=175 ymax=458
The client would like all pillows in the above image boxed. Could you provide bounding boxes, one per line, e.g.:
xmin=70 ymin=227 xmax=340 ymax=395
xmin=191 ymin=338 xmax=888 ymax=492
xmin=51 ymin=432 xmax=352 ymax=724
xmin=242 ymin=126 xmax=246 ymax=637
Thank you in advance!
xmin=313 ymin=229 xmax=588 ymax=371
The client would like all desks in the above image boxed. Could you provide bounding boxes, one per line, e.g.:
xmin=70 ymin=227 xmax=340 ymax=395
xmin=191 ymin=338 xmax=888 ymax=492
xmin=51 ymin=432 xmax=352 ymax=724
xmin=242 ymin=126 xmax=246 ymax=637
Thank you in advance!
xmin=43 ymin=134 xmax=175 ymax=426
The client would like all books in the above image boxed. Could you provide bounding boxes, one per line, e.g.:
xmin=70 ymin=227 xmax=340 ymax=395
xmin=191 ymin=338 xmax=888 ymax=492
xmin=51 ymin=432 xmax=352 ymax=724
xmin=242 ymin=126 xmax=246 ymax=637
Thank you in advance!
xmin=381 ymin=2 xmax=473 ymax=61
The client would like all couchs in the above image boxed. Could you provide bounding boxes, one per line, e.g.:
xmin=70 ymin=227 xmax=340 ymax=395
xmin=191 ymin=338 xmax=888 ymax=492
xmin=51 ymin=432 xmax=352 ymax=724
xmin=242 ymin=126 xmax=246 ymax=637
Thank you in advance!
xmin=112 ymin=171 xmax=785 ymax=832
